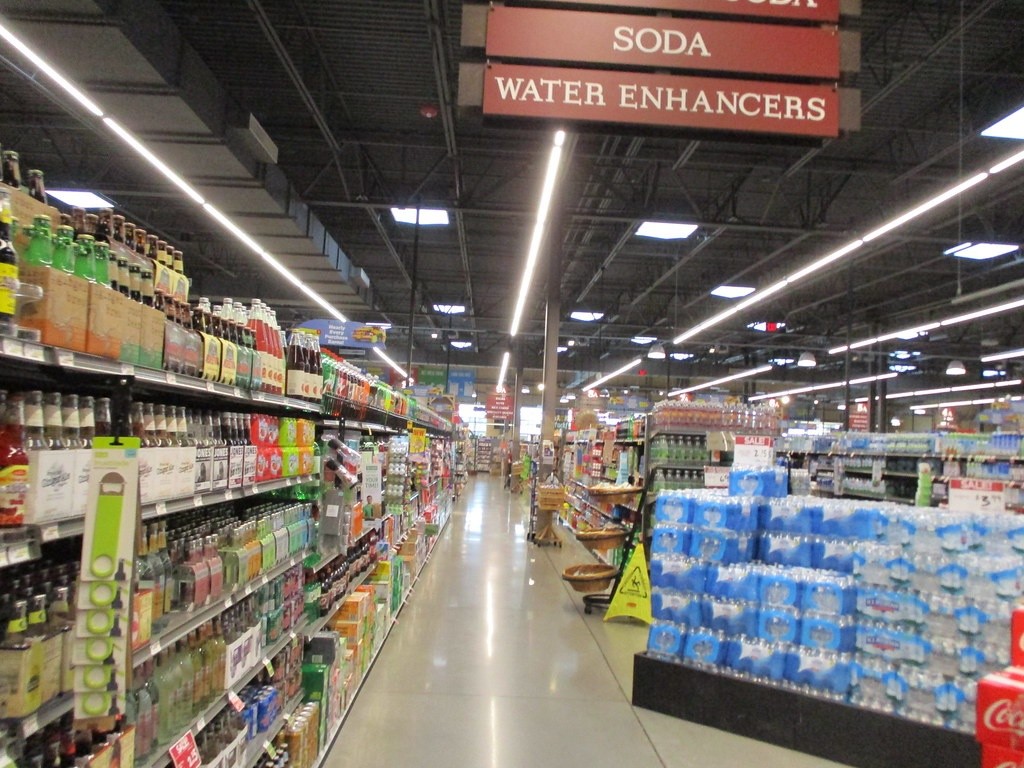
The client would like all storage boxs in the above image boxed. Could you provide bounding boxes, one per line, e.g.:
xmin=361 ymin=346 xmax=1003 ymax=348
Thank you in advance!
xmin=975 ymin=610 xmax=1024 ymax=768
xmin=21 ymin=447 xmax=197 ymax=529
xmin=580 ymin=430 xmax=589 ymax=440
xmin=19 ymin=259 xmax=169 ymax=373
xmin=511 ymin=463 xmax=524 ymax=475
xmin=301 ymin=476 xmax=455 ymax=748
xmin=599 ymin=430 xmax=615 ymax=440
xmin=535 ymin=484 xmax=564 ymax=512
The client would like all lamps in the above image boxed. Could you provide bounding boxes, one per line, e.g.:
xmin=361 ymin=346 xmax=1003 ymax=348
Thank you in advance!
xmin=813 ymin=381 xmax=846 ymax=394
xmin=647 ymin=340 xmax=666 ymax=360
xmin=495 ymin=130 xmax=577 ymax=404
xmin=582 ymin=357 xmax=641 ymax=397
xmin=0 ymin=22 xmax=416 ymax=386
xmin=668 ymin=363 xmax=773 ymax=398
xmin=797 ymin=351 xmax=816 ymax=368
xmin=747 ymin=387 xmax=813 ymax=403
xmin=945 ymin=359 xmax=967 ymax=376
xmin=673 ymin=147 xmax=1024 ymax=344
xmin=980 ymin=348 xmax=1024 ymax=363
xmin=849 ymin=373 xmax=1023 ymax=428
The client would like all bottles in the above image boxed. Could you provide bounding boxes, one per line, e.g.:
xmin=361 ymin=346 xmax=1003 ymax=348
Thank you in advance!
xmin=0 ymin=150 xmax=421 ymax=768
xmin=615 ymin=399 xmax=1023 ymax=735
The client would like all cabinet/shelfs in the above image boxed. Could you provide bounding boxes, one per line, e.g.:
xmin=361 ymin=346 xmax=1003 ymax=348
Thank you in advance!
xmin=453 ymin=423 xmax=470 ymax=503
xmin=386 ymin=410 xmax=430 ymax=639
xmin=466 ymin=437 xmax=476 ymax=472
xmin=476 ymin=438 xmax=491 ymax=472
xmin=642 ymin=413 xmax=784 ymax=540
xmin=0 ymin=333 xmax=326 ymax=768
xmin=930 ymin=453 xmax=1024 ymax=513
xmin=558 ymin=437 xmax=650 ymax=569
xmin=561 ymin=469 xmax=655 ymax=617
xmin=430 ymin=422 xmax=452 ymax=546
xmin=325 ymin=391 xmax=389 ymax=757
xmin=776 ymin=449 xmax=932 ymax=504
